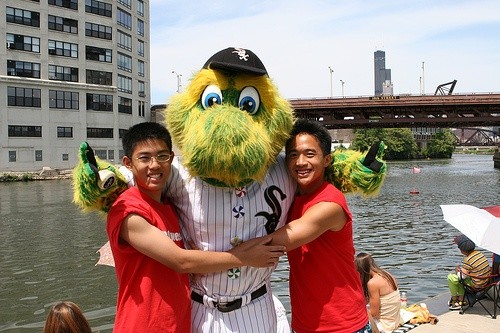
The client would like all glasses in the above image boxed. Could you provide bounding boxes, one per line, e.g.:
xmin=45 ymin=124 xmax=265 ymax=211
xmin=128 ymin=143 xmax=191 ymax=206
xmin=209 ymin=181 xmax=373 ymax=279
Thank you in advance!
xmin=135 ymin=153 xmax=172 ymax=163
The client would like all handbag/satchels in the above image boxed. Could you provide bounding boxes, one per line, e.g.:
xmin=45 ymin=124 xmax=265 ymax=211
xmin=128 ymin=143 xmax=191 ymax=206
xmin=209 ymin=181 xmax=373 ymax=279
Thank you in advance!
xmin=405 ymin=304 xmax=439 ymax=325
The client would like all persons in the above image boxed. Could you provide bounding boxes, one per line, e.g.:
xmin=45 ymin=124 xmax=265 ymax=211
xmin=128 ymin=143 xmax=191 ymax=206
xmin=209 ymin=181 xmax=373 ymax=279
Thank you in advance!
xmin=107 ymin=122 xmax=286 ymax=333
xmin=43 ymin=301 xmax=93 ymax=333
xmin=447 ymin=235 xmax=491 ymax=312
xmin=226 ymin=121 xmax=373 ymax=333
xmin=354 ymin=253 xmax=401 ymax=333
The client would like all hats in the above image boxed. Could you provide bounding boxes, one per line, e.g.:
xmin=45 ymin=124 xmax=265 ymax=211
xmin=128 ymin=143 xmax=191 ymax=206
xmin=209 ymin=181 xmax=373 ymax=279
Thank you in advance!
xmin=457 ymin=235 xmax=475 ymax=250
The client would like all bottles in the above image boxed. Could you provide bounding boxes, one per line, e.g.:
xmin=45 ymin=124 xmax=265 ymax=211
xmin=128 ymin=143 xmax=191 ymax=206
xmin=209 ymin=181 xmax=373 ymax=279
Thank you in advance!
xmin=400 ymin=292 xmax=407 ymax=307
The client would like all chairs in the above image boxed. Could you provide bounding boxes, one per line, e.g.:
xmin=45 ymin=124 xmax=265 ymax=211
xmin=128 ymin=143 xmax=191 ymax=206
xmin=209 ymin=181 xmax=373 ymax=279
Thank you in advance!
xmin=457 ymin=253 xmax=500 ymax=319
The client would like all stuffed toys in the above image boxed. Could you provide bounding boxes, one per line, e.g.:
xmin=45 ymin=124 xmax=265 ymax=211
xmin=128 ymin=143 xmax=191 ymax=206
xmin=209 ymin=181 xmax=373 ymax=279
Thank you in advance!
xmin=76 ymin=47 xmax=388 ymax=333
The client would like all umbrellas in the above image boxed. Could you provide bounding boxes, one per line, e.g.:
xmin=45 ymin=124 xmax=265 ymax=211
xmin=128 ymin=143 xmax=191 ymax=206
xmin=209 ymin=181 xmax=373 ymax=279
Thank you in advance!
xmin=439 ymin=202 xmax=500 ymax=254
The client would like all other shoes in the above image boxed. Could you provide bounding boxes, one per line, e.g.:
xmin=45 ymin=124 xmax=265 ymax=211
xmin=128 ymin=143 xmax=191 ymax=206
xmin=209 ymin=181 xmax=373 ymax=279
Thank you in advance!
xmin=448 ymin=297 xmax=469 ymax=310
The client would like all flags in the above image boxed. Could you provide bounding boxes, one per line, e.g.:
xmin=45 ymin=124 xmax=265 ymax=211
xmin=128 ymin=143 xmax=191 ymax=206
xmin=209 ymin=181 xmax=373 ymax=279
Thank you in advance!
xmin=413 ymin=166 xmax=421 ymax=174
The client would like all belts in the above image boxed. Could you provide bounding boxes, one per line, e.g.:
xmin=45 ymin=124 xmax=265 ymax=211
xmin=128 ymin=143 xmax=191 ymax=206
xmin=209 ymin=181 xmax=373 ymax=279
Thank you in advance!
xmin=191 ymin=284 xmax=267 ymax=313
